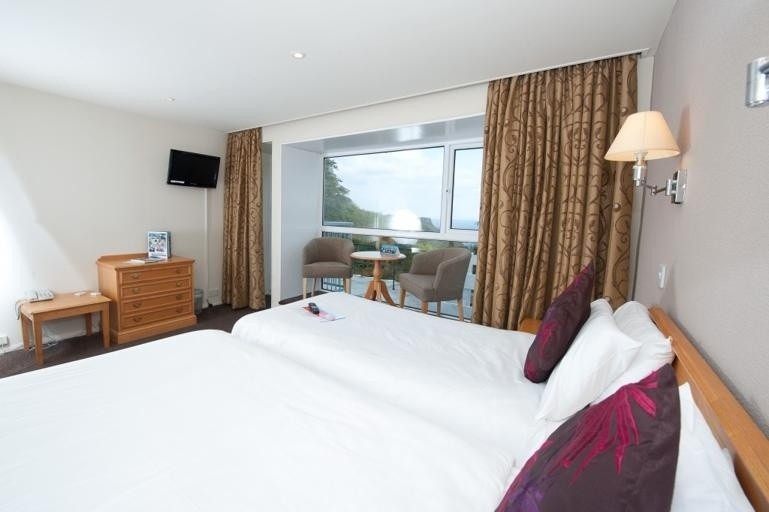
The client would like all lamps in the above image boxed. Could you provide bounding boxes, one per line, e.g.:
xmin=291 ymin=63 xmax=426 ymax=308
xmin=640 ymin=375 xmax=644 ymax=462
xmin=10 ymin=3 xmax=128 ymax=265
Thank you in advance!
xmin=604 ymin=111 xmax=688 ymax=204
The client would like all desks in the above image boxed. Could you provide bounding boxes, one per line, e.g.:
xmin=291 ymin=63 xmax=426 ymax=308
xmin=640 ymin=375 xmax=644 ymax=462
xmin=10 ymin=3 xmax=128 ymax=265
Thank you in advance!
xmin=20 ymin=291 xmax=113 ymax=365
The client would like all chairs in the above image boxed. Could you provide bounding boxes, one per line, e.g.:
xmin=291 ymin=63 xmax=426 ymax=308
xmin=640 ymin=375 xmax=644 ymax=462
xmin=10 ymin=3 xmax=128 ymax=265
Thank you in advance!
xmin=398 ymin=247 xmax=472 ymax=321
xmin=302 ymin=237 xmax=355 ymax=299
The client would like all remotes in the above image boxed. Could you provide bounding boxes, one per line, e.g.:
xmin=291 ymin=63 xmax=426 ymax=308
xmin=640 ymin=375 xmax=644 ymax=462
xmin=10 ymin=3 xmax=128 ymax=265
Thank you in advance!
xmin=309 ymin=302 xmax=321 ymax=314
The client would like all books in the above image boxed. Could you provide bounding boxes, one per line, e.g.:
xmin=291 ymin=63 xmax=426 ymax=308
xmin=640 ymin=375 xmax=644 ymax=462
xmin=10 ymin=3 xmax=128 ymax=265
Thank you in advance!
xmin=302 ymin=305 xmax=344 ymax=322
xmin=127 ymin=231 xmax=171 ymax=264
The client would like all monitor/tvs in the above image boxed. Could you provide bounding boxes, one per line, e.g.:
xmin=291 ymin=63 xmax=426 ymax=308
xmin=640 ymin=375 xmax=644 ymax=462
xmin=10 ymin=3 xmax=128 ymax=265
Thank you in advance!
xmin=166 ymin=148 xmax=221 ymax=189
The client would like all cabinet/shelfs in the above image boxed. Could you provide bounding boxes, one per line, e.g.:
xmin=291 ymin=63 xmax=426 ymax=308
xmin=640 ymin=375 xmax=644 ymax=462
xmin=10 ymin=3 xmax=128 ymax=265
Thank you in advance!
xmin=95 ymin=252 xmax=198 ymax=345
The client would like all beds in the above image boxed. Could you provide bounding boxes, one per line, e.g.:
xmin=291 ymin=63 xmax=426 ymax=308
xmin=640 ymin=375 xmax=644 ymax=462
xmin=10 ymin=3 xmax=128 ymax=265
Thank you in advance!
xmin=230 ymin=291 xmax=547 ymax=469
xmin=0 ymin=328 xmax=521 ymax=511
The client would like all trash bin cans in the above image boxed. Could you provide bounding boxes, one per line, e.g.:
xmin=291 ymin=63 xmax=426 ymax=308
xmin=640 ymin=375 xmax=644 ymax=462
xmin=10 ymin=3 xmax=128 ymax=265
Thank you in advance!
xmin=195 ymin=289 xmax=203 ymax=315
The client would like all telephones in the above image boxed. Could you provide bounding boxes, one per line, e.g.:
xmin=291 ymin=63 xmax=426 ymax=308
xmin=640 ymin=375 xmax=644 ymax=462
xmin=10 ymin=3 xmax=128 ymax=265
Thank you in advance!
xmin=25 ymin=288 xmax=54 ymax=303
